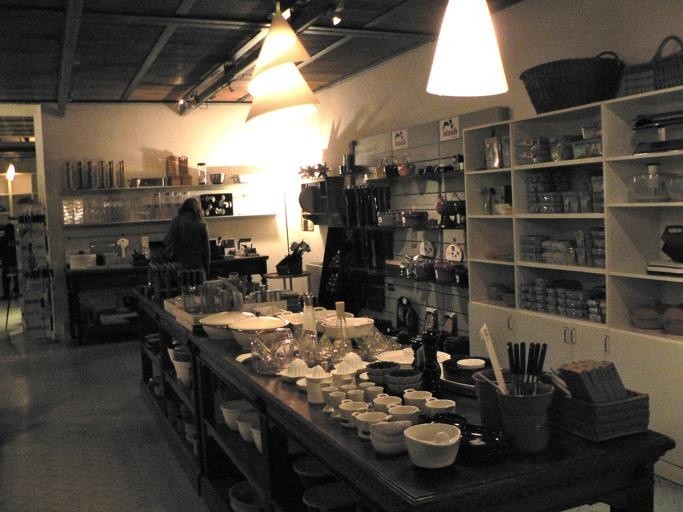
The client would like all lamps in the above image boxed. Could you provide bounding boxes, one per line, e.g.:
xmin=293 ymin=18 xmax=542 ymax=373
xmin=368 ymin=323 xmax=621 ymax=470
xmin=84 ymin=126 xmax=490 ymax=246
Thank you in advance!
xmin=425 ymin=0 xmax=509 ymax=99
xmin=244 ymin=1 xmax=321 ymax=123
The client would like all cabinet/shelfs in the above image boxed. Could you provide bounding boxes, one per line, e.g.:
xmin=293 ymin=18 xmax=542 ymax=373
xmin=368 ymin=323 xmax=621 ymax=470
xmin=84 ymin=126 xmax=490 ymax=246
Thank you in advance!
xmin=600 ymin=327 xmax=683 ymax=491
xmin=601 ymin=84 xmax=683 ymax=341
xmin=467 ymin=301 xmax=514 ymax=370
xmin=298 ymin=177 xmax=343 ymax=221
xmin=131 ymin=285 xmax=206 ymax=498
xmin=192 ymin=336 xmax=676 ymax=511
xmin=61 ymin=180 xmax=278 ymax=229
xmin=511 ymin=101 xmax=607 ymax=327
xmin=514 ymin=311 xmax=602 ymax=383
xmin=461 ymin=119 xmax=516 ymax=307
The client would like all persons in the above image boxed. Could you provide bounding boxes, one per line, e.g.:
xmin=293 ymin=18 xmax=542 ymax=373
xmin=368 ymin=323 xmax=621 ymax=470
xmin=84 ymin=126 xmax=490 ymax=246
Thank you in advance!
xmin=162 ymin=196 xmax=211 ymax=269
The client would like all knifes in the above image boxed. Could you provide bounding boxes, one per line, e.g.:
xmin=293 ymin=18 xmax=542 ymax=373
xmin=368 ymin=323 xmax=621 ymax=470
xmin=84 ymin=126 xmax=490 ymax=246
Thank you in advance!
xmin=506 ymin=342 xmax=513 ymax=395
xmin=539 ymin=343 xmax=547 ymax=380
xmin=512 ymin=343 xmax=519 ymax=394
xmin=533 ymin=357 xmax=540 ymax=394
xmin=519 ymin=342 xmax=526 ymax=395
xmin=527 ymin=342 xmax=535 ymax=395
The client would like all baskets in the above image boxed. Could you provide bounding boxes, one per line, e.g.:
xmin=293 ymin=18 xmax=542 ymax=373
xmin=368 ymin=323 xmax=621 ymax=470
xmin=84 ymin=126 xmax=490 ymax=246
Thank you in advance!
xmin=519 ymin=51 xmax=624 ymax=114
xmin=624 ymin=35 xmax=683 ymax=96
xmin=547 ymin=389 xmax=650 ymax=442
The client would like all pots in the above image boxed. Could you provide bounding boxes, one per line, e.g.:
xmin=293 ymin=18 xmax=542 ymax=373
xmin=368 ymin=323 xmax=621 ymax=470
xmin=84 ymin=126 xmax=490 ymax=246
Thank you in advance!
xmin=377 ymin=211 xmax=396 ymax=226
xmin=434 ymin=262 xmax=463 ymax=284
xmin=412 ymin=256 xmax=435 ymax=281
xmin=401 ymin=211 xmax=428 ymax=226
xmin=630 ymin=301 xmax=682 ymax=336
xmin=167 ymin=344 xmax=190 ymax=368
xmin=396 ymin=210 xmax=402 ymax=226
xmin=173 ymin=347 xmax=217 ymax=386
xmin=385 ymin=165 xmax=399 ymax=177
xmin=397 ymin=165 xmax=414 ymax=176
xmin=398 ymin=256 xmax=411 ymax=280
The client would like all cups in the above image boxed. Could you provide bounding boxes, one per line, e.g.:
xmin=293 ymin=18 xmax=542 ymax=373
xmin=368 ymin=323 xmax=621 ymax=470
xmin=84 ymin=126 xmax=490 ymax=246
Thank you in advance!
xmin=151 ymin=191 xmax=190 ymax=219
xmin=359 ymin=381 xmax=374 ymax=390
xmin=496 ymin=381 xmax=555 ymax=453
xmin=340 ymin=384 xmax=357 ymax=392
xmin=471 ymin=369 xmax=509 ymax=433
xmin=320 ymin=385 xmax=337 ymax=412
xmin=329 ymin=391 xmax=346 ymax=417
xmin=361 ymin=325 xmax=401 ymax=362
xmin=249 ymin=328 xmax=335 ymax=376
xmin=257 ymin=284 xmax=283 ymax=304
xmin=366 ymin=386 xmax=384 ymax=400
xmin=349 ymin=389 xmax=364 ymax=401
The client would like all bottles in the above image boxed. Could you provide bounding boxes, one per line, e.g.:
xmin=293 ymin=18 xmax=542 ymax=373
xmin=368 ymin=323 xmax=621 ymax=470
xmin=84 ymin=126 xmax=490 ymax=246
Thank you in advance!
xmin=298 ymin=290 xmax=318 ymax=353
xmin=196 ymin=162 xmax=206 ymax=184
xmin=332 ymin=301 xmax=352 ymax=352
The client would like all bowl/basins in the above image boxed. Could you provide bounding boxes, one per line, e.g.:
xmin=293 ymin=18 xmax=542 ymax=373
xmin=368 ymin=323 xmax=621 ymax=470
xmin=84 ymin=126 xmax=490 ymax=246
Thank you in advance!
xmin=197 ymin=311 xmax=257 ymax=341
xmin=226 ymin=315 xmax=289 ymax=351
xmin=372 ymin=393 xmax=402 ymax=410
xmin=218 ymin=399 xmax=252 ymax=432
xmin=424 ymin=397 xmax=456 ymax=416
xmin=351 ymin=411 xmax=392 ymax=440
xmin=367 ymin=420 xmax=412 ymax=455
xmin=402 ymin=388 xmax=433 ymax=407
xmin=233 ymin=412 xmax=260 ymax=444
xmin=366 ymin=361 xmax=400 ymax=385
xmin=665 ymin=174 xmax=683 ymax=202
xmin=620 ymin=172 xmax=669 ymax=202
xmin=387 ymin=403 xmax=423 ymax=424
xmin=339 ymin=399 xmax=368 ymax=428
xmin=402 ymin=423 xmax=462 ymax=469
xmin=442 ymin=356 xmax=491 ymax=388
xmin=385 ymin=368 xmax=423 ymax=393
xmin=208 ymin=173 xmax=225 ymax=184
xmin=276 ymin=306 xmax=374 ymax=338
xmin=248 ymin=420 xmax=262 ymax=457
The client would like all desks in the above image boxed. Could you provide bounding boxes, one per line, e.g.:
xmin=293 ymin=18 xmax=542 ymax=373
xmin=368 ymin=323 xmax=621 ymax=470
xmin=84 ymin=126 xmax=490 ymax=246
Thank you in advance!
xmin=66 ymin=263 xmax=150 ymax=346
xmin=209 ymin=254 xmax=269 ymax=289
xmin=264 ymin=271 xmax=312 ymax=294
